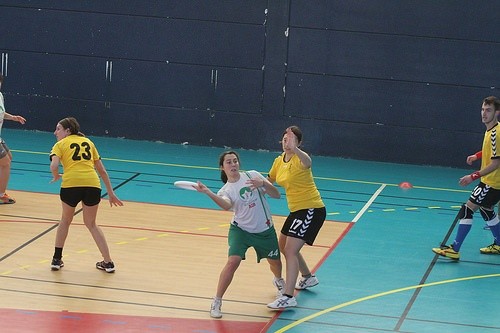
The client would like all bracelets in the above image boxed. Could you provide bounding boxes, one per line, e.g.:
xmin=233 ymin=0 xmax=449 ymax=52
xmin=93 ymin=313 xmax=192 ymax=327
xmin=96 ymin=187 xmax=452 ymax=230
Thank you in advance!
xmin=470 ymin=171 xmax=481 ymax=180
xmin=474 ymin=151 xmax=482 ymax=159
xmin=263 ymin=180 xmax=265 ymax=187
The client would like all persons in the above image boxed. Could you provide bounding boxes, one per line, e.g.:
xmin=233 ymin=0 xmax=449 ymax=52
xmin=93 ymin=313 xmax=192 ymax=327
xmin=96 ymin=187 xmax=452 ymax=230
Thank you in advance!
xmin=0 ymin=74 xmax=26 ymax=204
xmin=48 ymin=117 xmax=125 ymax=273
xmin=262 ymin=126 xmax=327 ymax=309
xmin=432 ymin=95 xmax=500 ymax=261
xmin=191 ymin=150 xmax=287 ymax=318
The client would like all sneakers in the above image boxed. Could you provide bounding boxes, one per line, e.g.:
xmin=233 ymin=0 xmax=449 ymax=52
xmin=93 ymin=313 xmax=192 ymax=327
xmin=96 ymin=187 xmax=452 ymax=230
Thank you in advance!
xmin=271 ymin=277 xmax=286 ymax=291
xmin=480 ymin=240 xmax=500 ymax=255
xmin=267 ymin=295 xmax=298 ymax=309
xmin=295 ymin=274 xmax=318 ymax=289
xmin=96 ymin=260 xmax=116 ymax=272
xmin=210 ymin=298 xmax=222 ymax=318
xmin=0 ymin=193 xmax=16 ymax=204
xmin=432 ymin=244 xmax=459 ymax=260
xmin=51 ymin=256 xmax=63 ymax=270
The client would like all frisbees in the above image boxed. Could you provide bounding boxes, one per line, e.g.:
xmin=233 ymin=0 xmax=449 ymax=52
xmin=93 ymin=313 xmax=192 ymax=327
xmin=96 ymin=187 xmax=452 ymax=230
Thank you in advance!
xmin=173 ymin=180 xmax=201 ymax=192
